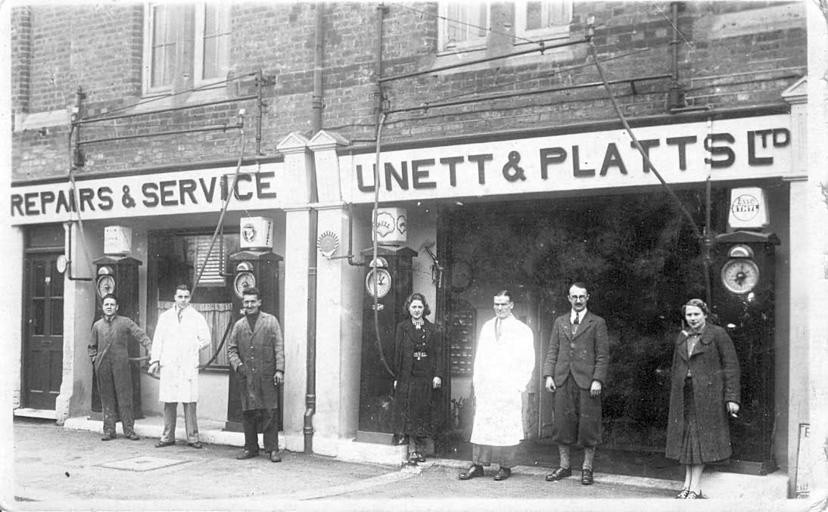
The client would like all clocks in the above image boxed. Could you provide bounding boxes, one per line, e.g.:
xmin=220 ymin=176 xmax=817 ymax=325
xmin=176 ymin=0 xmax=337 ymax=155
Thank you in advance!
xmin=713 ymin=231 xmax=782 ymax=462
xmin=91 ymin=253 xmax=145 ymax=419
xmin=358 ymin=244 xmax=419 ymax=433
xmin=226 ymin=248 xmax=283 ymax=424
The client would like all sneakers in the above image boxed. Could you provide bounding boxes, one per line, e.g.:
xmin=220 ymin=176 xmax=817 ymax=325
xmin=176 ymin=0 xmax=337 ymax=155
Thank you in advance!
xmin=128 ymin=433 xmax=139 ymax=440
xmin=190 ymin=441 xmax=202 ymax=447
xmin=102 ymin=434 xmax=116 ymax=440
xmin=155 ymin=441 xmax=175 ymax=447
xmin=270 ymin=451 xmax=280 ymax=462
xmin=237 ymin=450 xmax=259 ymax=458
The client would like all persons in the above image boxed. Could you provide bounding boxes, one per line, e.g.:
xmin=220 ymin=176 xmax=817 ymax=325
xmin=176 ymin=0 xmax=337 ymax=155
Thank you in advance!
xmin=88 ymin=294 xmax=152 ymax=441
xmin=544 ymin=282 xmax=610 ymax=485
xmin=458 ymin=290 xmax=536 ymax=479
xmin=665 ymin=299 xmax=741 ymax=499
xmin=393 ymin=294 xmax=443 ymax=465
xmin=228 ymin=287 xmax=284 ymax=462
xmin=148 ymin=284 xmax=210 ymax=449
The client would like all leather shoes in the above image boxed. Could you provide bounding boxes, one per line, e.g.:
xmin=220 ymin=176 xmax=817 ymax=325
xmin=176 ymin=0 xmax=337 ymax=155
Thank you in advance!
xmin=677 ymin=489 xmax=702 ymax=500
xmin=546 ymin=465 xmax=572 ymax=481
xmin=408 ymin=449 xmax=424 ymax=464
xmin=582 ymin=469 xmax=593 ymax=485
xmin=459 ymin=465 xmax=483 ymax=479
xmin=495 ymin=466 xmax=511 ymax=480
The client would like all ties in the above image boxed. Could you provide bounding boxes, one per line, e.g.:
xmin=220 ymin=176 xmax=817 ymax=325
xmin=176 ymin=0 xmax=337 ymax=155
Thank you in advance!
xmin=573 ymin=313 xmax=579 ymax=326
